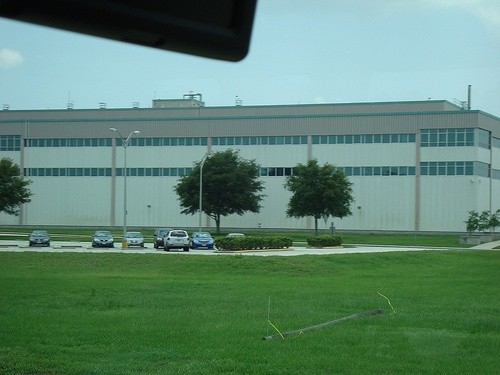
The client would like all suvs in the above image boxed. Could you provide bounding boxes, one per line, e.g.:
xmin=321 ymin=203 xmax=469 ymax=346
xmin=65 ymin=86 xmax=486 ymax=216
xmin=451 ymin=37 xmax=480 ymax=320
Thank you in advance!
xmin=154 ymin=229 xmax=171 ymax=248
xmin=163 ymin=229 xmax=190 ymax=252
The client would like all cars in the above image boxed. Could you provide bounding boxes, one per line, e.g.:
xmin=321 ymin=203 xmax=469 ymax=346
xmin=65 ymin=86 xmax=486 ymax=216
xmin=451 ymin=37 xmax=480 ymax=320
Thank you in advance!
xmin=227 ymin=234 xmax=245 ymax=238
xmin=122 ymin=232 xmax=145 ymax=248
xmin=91 ymin=231 xmax=115 ymax=248
xmin=28 ymin=230 xmax=52 ymax=247
xmin=190 ymin=232 xmax=215 ymax=250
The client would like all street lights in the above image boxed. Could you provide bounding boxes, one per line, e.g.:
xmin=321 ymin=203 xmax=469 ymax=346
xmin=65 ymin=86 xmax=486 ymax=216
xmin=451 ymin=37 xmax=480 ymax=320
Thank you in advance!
xmin=109 ymin=127 xmax=140 ymax=250
